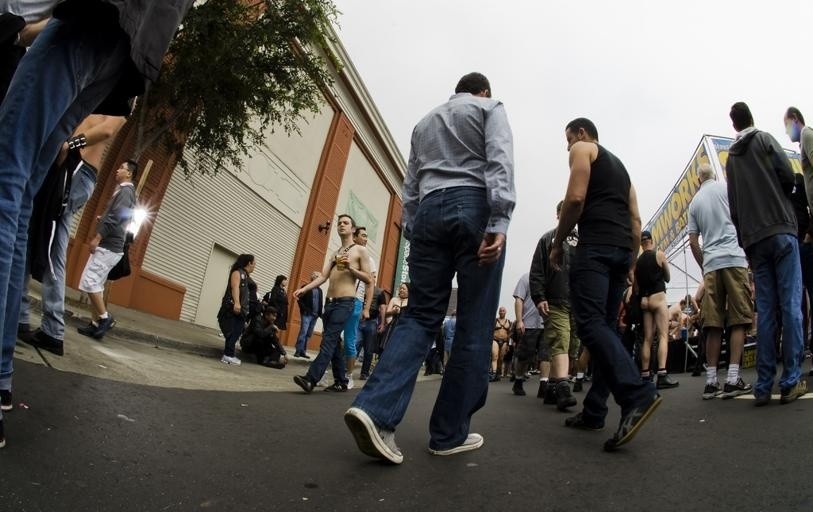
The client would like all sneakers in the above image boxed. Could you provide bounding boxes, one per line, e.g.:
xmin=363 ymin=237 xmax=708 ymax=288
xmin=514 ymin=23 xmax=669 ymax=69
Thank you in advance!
xmin=512 ymin=378 xmax=527 ymax=396
xmin=344 ymin=406 xmax=405 ymax=465
xmin=703 ymin=380 xmax=723 ymax=399
xmin=18 ymin=323 xmax=64 ymax=356
xmin=428 ymin=433 xmax=484 ymax=456
xmin=294 ymin=373 xmax=314 ymax=393
xmin=538 ymin=378 xmax=558 ymax=406
xmin=221 ymin=355 xmax=241 ymax=366
xmin=604 ymin=383 xmax=664 ymax=448
xmin=324 ymin=374 xmax=354 ymax=393
xmin=0 ymin=391 xmax=13 ymax=448
xmin=315 ymin=371 xmax=330 ymax=387
xmin=93 ymin=312 xmax=116 ymax=337
xmin=78 ymin=320 xmax=102 ymax=340
xmin=566 ymin=408 xmax=606 ymax=430
xmin=690 ymin=372 xmax=701 ymax=377
xmin=722 ymin=378 xmax=753 ymax=398
xmin=573 ymin=377 xmax=584 ymax=393
xmin=555 ymin=383 xmax=577 ymax=410
xmin=755 ymin=389 xmax=773 ymax=406
xmin=781 ymin=373 xmax=805 ymax=402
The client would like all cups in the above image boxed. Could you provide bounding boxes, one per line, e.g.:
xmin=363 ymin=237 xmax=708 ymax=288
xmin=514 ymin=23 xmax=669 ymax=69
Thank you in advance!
xmin=336 ymin=253 xmax=347 ymax=271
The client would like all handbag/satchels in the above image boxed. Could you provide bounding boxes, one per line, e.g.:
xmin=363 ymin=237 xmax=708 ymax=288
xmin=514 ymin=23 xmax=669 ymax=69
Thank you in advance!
xmin=106 ymin=237 xmax=133 ymax=280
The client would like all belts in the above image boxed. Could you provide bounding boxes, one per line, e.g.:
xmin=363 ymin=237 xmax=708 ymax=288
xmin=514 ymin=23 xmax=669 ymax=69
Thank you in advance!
xmin=326 ymin=296 xmax=355 ymax=303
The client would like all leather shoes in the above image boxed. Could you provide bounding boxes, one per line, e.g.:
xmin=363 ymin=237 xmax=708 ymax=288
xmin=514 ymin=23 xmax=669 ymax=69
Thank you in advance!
xmin=294 ymin=350 xmax=310 ymax=359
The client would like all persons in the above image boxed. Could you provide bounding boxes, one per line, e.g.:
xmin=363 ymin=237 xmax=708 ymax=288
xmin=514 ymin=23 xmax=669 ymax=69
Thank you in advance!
xmin=0 ymin=1 xmax=813 ymax=466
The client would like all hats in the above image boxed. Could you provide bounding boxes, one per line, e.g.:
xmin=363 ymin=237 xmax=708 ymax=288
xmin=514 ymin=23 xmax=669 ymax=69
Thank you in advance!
xmin=641 ymin=230 xmax=652 ymax=242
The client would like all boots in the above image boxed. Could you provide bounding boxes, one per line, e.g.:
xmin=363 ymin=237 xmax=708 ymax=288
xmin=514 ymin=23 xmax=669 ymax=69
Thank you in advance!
xmin=640 ymin=367 xmax=680 ymax=390
